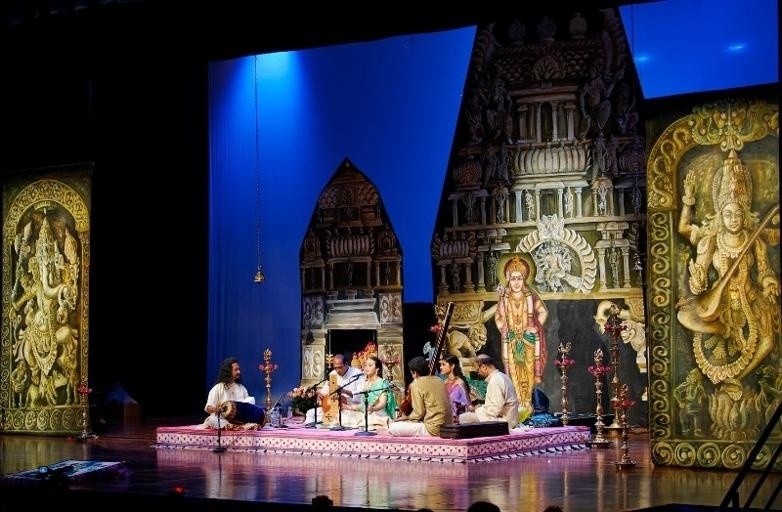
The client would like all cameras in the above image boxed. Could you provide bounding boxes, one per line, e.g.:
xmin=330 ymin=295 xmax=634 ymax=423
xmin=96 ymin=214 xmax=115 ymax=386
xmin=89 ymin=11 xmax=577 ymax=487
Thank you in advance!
xmin=38 ymin=466 xmax=53 ymax=474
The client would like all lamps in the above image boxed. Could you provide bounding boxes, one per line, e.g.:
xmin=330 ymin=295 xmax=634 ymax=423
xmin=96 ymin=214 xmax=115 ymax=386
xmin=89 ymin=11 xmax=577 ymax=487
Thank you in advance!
xmin=254 ymin=54 xmax=265 ymax=282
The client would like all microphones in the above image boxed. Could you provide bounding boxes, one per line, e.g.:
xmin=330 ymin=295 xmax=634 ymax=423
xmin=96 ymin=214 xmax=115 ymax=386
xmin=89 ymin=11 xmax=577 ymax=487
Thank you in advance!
xmin=326 ymin=367 xmax=334 ymax=375
xmin=217 ymin=406 xmax=223 ymax=413
xmin=391 ymin=382 xmax=405 ymax=392
xmin=353 ymin=373 xmax=364 ymax=377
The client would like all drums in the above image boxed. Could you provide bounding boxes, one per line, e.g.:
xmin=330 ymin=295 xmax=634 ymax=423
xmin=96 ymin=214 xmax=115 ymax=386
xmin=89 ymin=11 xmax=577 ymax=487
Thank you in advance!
xmin=220 ymin=400 xmax=268 ymax=426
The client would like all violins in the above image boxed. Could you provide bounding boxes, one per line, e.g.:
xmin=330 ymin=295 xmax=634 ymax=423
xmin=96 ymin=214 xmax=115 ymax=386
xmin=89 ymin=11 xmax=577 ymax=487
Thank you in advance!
xmin=399 ymin=383 xmax=413 ymax=416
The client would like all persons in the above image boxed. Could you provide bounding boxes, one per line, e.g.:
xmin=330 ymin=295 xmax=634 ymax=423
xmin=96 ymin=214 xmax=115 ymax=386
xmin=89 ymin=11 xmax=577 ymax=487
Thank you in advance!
xmin=10 ymin=360 xmax=27 ymax=407
xmin=494 ymin=256 xmax=549 ymax=415
xmin=677 ymin=151 xmax=782 ymax=391
xmin=457 ymin=354 xmax=519 ymax=430
xmin=673 ymin=368 xmax=707 ymax=436
xmin=203 ymin=357 xmax=263 ymax=432
xmin=65 ymin=355 xmax=79 ymax=403
xmin=598 ymin=185 xmax=608 ymax=215
xmin=496 ymin=191 xmax=506 ymax=224
xmin=545 ymin=247 xmax=564 ymax=292
xmin=544 ymin=505 xmax=563 ymax=512
xmin=440 ymin=354 xmax=471 ymax=424
xmin=467 ymin=501 xmax=500 ymax=512
xmin=389 ymin=356 xmax=453 ymax=436
xmin=336 ymin=357 xmax=396 ymax=431
xmin=303 ymin=354 xmax=366 ymax=428
